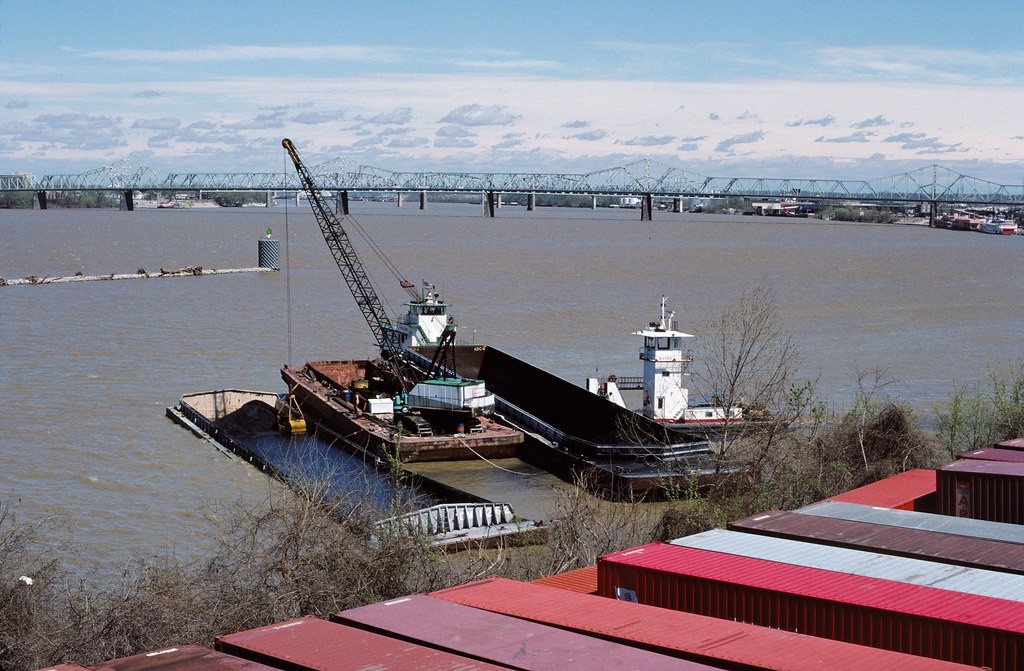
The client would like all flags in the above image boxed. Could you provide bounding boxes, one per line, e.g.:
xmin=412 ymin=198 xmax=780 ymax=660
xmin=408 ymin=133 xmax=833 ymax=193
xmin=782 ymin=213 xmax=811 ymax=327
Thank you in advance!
xmin=423 ymin=281 xmax=435 ymax=291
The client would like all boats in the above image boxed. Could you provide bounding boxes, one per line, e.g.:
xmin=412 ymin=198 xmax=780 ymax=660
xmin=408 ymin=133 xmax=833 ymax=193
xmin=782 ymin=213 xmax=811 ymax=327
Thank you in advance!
xmin=981 ymin=215 xmax=1017 ymax=235
xmin=157 ymin=202 xmax=174 ymax=208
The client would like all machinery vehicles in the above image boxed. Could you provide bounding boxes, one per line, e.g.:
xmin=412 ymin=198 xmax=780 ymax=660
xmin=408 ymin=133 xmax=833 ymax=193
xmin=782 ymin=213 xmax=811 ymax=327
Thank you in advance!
xmin=276 ymin=138 xmax=495 ymax=436
